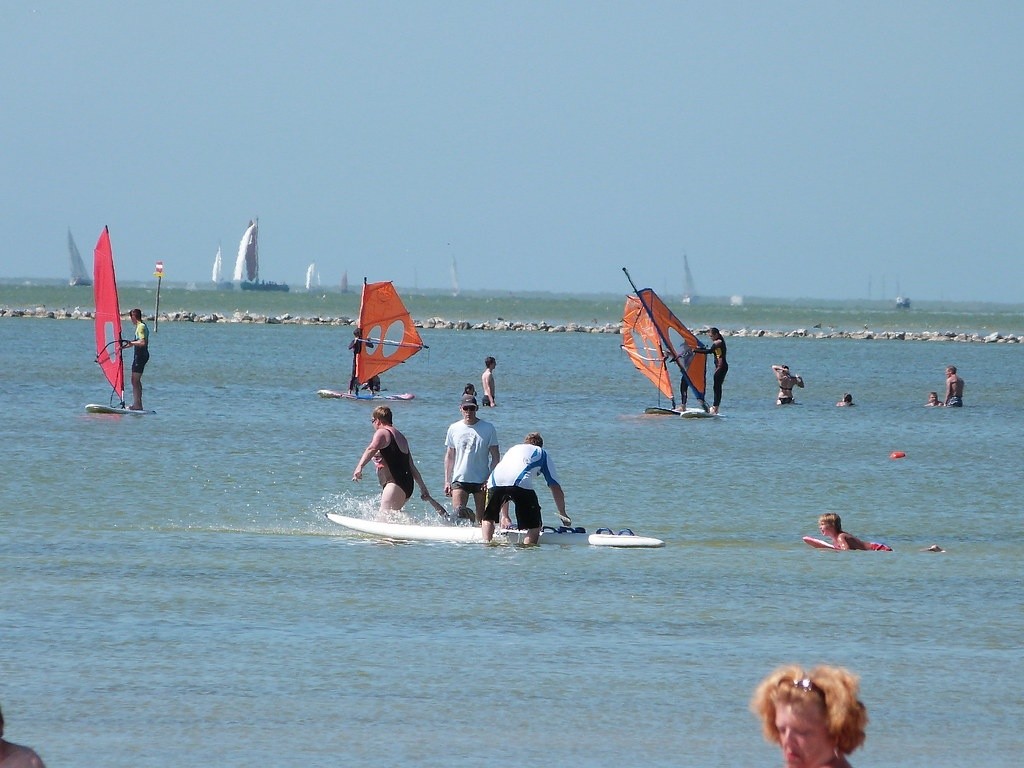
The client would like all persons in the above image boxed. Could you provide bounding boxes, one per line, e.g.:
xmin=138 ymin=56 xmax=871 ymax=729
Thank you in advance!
xmin=481 ymin=432 xmax=570 ymax=546
xmin=673 ymin=328 xmax=706 ymax=412
xmin=348 ymin=328 xmax=374 ymax=396
xmin=0 ymin=707 xmax=45 ymax=768
xmin=444 ymin=398 xmax=511 ymax=529
xmin=772 ymin=365 xmax=805 ymax=405
xmin=944 ymin=366 xmax=964 ymax=407
xmin=351 ymin=406 xmax=429 ymax=513
xmin=125 ymin=309 xmax=149 ymax=410
xmin=691 ymin=328 xmax=728 ymax=415
xmin=924 ymin=392 xmax=943 ymax=406
xmin=753 ymin=663 xmax=868 ymax=768
xmin=462 ymin=382 xmax=477 ymax=397
xmin=837 ymin=393 xmax=853 ymax=406
xmin=818 ymin=513 xmax=941 ymax=552
xmin=482 ymin=357 xmax=497 ymax=407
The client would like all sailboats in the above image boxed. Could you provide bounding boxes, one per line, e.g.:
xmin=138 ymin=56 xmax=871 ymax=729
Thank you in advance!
xmin=64 ymin=227 xmax=94 ymax=288
xmin=210 ymin=214 xmax=291 ymax=293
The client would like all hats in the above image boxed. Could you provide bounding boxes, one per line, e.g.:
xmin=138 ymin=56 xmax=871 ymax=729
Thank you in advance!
xmin=461 ymin=395 xmax=477 ymax=406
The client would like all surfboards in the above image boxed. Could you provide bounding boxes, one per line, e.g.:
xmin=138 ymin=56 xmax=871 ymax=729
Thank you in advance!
xmin=644 ymin=406 xmax=727 ymax=419
xmin=85 ymin=403 xmax=157 ymax=414
xmin=325 ymin=511 xmax=666 ymax=549
xmin=801 ymin=535 xmax=946 ymax=553
xmin=317 ymin=390 xmax=416 ymax=401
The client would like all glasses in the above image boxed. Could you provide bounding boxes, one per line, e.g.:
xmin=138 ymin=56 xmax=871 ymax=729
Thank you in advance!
xmin=372 ymin=418 xmax=377 ymax=423
xmin=778 ymin=678 xmax=825 ymax=700
xmin=462 ymin=406 xmax=475 ymax=411
